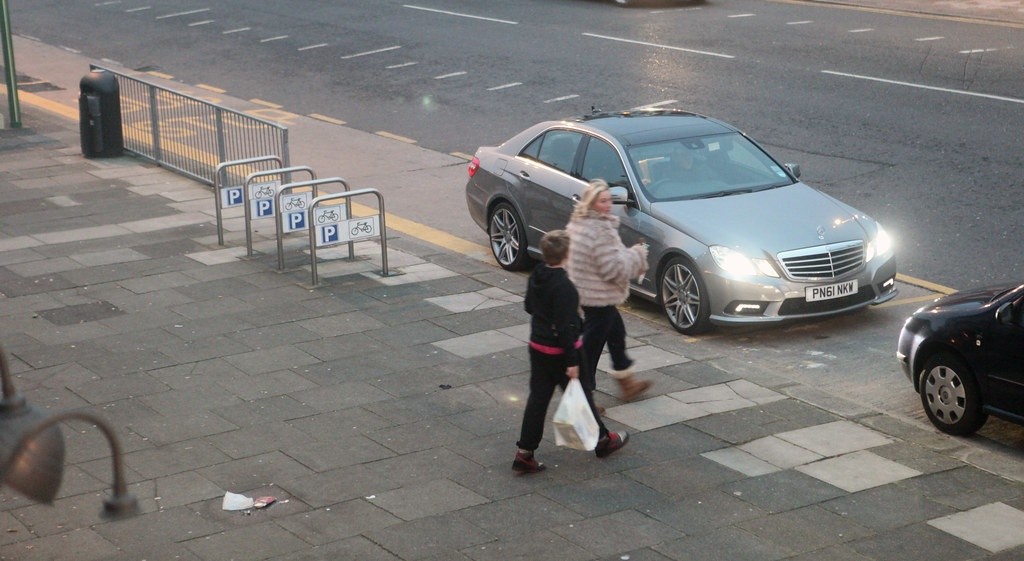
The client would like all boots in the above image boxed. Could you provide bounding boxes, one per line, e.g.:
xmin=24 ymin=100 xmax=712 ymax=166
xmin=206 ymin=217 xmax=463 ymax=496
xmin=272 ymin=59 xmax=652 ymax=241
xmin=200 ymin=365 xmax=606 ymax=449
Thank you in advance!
xmin=611 ymin=359 xmax=654 ymax=401
xmin=595 ymin=404 xmax=607 ymax=417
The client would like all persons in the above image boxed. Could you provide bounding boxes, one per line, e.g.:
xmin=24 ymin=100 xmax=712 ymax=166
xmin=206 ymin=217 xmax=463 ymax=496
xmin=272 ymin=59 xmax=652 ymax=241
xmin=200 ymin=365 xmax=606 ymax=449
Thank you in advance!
xmin=656 ymin=144 xmax=719 ymax=204
xmin=510 ymin=230 xmax=630 ymax=472
xmin=563 ymin=178 xmax=655 ymax=416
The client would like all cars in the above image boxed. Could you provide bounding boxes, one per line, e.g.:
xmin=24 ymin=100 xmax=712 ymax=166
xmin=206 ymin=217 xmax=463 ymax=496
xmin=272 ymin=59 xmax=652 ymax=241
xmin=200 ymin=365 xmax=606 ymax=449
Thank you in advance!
xmin=894 ymin=278 xmax=1024 ymax=437
xmin=464 ymin=102 xmax=899 ymax=337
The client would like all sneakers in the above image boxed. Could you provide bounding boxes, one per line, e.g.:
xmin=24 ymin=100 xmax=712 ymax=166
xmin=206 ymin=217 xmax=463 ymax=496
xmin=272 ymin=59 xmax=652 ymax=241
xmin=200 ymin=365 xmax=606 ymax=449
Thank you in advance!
xmin=595 ymin=430 xmax=629 ymax=458
xmin=512 ymin=450 xmax=546 ymax=474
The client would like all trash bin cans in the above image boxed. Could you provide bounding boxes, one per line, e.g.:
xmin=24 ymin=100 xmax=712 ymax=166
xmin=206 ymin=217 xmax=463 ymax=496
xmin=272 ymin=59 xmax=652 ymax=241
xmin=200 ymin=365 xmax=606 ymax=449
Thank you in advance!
xmin=79 ymin=69 xmax=124 ymax=158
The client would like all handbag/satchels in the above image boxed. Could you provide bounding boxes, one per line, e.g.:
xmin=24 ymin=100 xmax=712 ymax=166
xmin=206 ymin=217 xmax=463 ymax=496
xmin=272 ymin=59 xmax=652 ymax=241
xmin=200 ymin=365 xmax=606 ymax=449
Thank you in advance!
xmin=552 ymin=377 xmax=600 ymax=451
